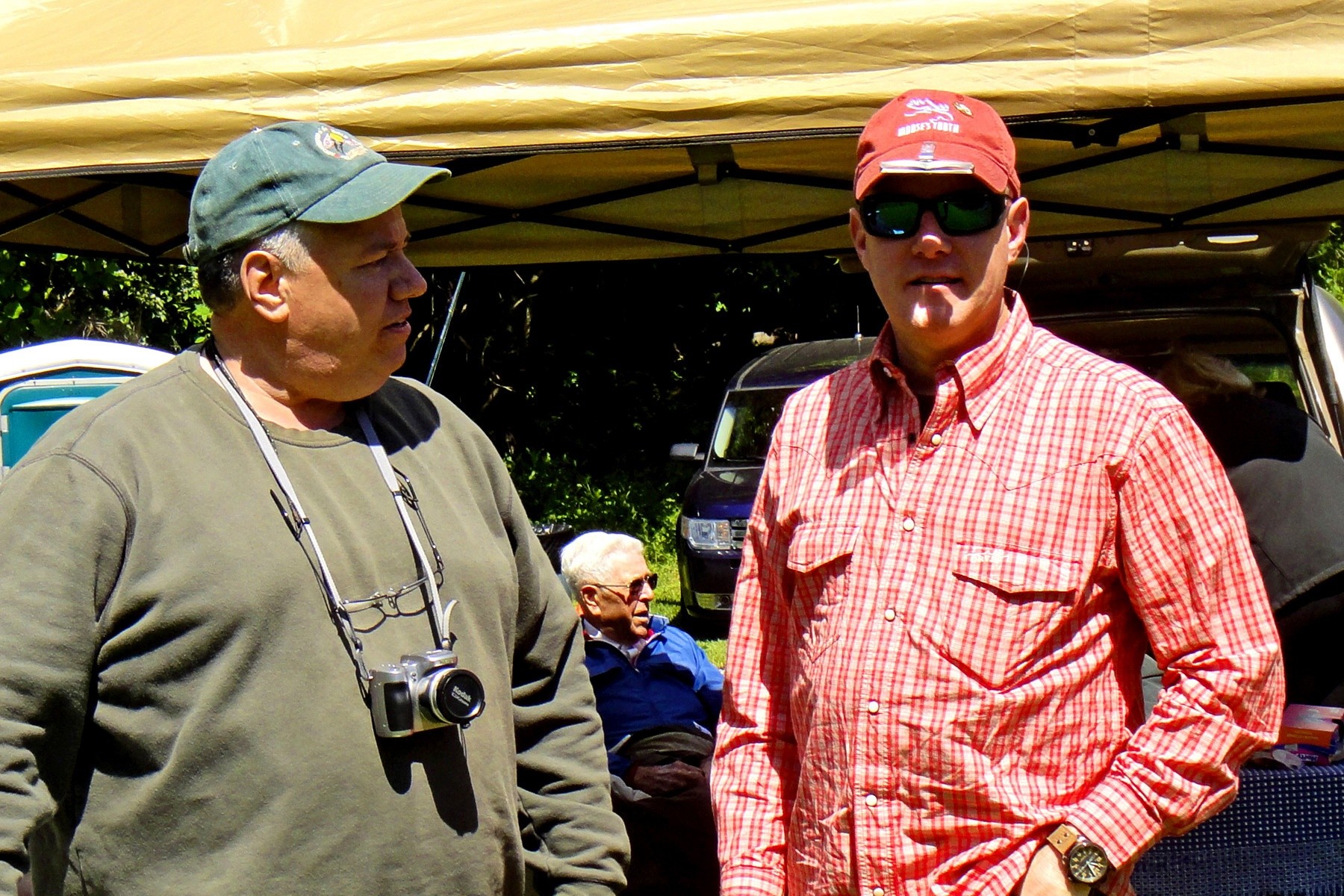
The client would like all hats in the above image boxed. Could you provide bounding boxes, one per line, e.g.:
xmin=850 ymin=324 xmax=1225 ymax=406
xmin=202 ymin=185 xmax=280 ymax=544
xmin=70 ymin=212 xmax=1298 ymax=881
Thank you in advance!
xmin=852 ymin=89 xmax=1021 ymax=205
xmin=182 ymin=122 xmax=452 ymax=269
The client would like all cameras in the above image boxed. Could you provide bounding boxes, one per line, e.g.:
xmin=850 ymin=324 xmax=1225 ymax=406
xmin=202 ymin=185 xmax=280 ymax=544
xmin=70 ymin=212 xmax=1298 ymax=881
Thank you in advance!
xmin=369 ymin=649 xmax=486 ymax=737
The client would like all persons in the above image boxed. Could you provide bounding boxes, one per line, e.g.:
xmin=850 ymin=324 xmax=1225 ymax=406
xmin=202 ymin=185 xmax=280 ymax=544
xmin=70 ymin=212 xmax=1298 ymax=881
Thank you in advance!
xmin=710 ymin=89 xmax=1285 ymax=896
xmin=0 ymin=123 xmax=633 ymax=896
xmin=1154 ymin=347 xmax=1344 ymax=706
xmin=561 ymin=531 xmax=724 ymax=896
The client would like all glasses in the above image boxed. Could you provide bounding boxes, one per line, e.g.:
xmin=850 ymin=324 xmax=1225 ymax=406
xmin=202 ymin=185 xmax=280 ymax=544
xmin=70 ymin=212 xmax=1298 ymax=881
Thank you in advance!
xmin=859 ymin=186 xmax=1006 ymax=241
xmin=594 ymin=573 xmax=659 ymax=598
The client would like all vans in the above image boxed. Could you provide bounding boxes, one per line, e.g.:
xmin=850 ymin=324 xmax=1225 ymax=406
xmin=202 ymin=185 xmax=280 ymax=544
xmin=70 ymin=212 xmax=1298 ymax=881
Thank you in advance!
xmin=1002 ymin=219 xmax=1344 ymax=467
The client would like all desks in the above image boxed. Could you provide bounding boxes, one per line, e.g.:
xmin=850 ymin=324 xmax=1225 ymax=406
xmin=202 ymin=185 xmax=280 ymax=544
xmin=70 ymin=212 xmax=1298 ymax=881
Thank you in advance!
xmin=1129 ymin=758 xmax=1344 ymax=896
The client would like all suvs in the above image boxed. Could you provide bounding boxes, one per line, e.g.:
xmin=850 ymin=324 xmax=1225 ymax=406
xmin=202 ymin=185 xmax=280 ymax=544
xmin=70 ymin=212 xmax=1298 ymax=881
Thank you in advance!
xmin=667 ymin=335 xmax=877 ymax=627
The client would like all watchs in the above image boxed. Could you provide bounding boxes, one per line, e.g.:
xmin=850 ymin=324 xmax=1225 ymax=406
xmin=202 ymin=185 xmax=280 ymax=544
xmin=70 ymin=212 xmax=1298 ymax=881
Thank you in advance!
xmin=1046 ymin=824 xmax=1109 ymax=886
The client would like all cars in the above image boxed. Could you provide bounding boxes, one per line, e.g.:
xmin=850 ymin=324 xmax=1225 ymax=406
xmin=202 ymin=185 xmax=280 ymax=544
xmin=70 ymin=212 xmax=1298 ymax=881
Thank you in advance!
xmin=0 ymin=334 xmax=177 ymax=471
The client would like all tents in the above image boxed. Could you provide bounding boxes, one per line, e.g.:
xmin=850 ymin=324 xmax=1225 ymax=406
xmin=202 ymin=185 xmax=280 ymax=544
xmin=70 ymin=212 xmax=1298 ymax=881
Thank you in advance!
xmin=0 ymin=0 xmax=1344 ymax=261
